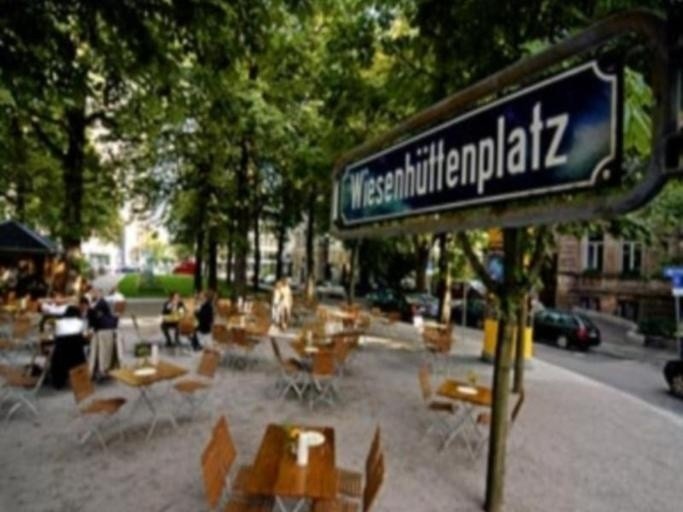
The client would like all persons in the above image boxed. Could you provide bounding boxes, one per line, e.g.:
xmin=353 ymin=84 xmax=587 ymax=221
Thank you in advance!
xmin=269 ymin=280 xmax=286 ymax=334
xmin=280 ymin=277 xmax=294 ymax=325
xmin=187 ymin=290 xmax=214 ymax=353
xmin=0 ymin=249 xmax=118 ymax=392
xmin=411 ymin=302 xmax=427 ymax=334
xmin=160 ymin=291 xmax=187 ymax=347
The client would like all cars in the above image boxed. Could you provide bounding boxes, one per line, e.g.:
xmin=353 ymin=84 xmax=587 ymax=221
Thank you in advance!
xmin=660 ymin=357 xmax=683 ymax=403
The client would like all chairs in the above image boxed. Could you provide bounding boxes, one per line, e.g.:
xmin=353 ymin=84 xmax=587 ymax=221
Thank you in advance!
xmin=0 ymin=289 xmax=220 ymax=452
xmin=371 ymin=302 xmax=456 ymax=362
xmin=415 ymin=365 xmax=525 ymax=464
xmin=166 ymin=290 xmax=372 ymax=414
xmin=199 ymin=416 xmax=385 ymax=512
